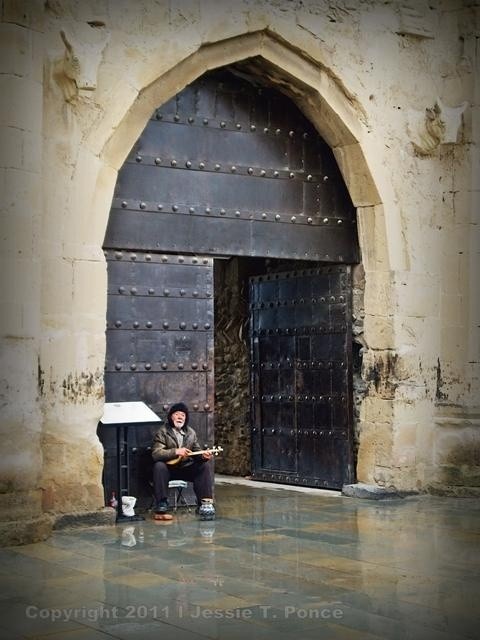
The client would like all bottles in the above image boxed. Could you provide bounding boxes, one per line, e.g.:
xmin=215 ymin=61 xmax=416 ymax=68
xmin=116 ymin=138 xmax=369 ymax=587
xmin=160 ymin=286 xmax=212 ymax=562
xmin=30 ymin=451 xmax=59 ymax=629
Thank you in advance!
xmin=109 ymin=492 xmax=119 ymax=524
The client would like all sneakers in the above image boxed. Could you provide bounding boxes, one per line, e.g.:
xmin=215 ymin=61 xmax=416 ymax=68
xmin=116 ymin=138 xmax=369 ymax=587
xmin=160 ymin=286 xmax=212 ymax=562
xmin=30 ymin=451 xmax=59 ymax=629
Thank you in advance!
xmin=155 ymin=502 xmax=168 ymax=514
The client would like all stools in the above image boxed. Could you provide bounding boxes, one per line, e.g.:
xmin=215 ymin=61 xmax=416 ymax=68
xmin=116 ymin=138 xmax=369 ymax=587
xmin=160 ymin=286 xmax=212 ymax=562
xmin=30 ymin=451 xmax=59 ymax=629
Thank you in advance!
xmin=149 ymin=481 xmax=192 ymax=515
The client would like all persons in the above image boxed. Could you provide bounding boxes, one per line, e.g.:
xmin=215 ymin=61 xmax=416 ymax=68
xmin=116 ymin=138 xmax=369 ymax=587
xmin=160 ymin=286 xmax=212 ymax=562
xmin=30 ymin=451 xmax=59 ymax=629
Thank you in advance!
xmin=147 ymin=401 xmax=216 ymax=515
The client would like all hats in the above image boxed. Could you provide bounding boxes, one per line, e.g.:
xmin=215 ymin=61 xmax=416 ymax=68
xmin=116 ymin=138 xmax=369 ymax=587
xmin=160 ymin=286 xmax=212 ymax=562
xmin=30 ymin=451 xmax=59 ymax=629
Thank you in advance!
xmin=168 ymin=402 xmax=190 ymax=430
